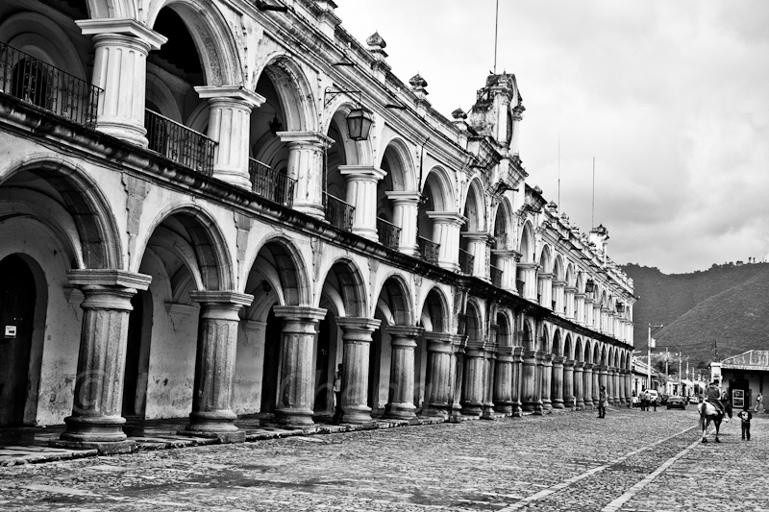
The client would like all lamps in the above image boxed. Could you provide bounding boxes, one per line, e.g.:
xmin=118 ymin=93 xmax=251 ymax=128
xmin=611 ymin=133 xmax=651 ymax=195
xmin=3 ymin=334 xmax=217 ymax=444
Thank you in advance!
xmin=323 ymin=84 xmax=373 ymax=142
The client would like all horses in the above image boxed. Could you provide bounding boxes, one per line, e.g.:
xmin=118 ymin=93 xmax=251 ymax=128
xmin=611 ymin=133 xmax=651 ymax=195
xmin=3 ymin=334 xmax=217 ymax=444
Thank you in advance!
xmin=698 ymin=395 xmax=733 ymax=444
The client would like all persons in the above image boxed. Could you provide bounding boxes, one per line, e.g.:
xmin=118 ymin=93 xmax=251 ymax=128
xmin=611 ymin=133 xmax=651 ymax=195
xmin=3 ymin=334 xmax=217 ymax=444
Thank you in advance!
xmin=707 ymin=379 xmax=760 ymax=441
xmin=332 ymin=363 xmax=342 ymax=407
xmin=597 ymin=386 xmax=605 ymax=418
xmin=631 ymin=389 xmax=652 ymax=411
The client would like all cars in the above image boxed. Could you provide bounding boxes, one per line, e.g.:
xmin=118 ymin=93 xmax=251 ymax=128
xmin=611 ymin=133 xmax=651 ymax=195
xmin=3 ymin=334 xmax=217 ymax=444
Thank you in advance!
xmin=633 ymin=389 xmax=668 ymax=406
xmin=666 ymin=396 xmax=686 ymax=410
xmin=689 ymin=397 xmax=699 ymax=404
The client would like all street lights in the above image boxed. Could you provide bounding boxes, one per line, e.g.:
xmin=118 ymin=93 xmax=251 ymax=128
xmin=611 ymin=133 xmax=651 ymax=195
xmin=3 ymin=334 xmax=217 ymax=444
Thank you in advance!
xmin=648 ymin=320 xmax=663 ymax=389
